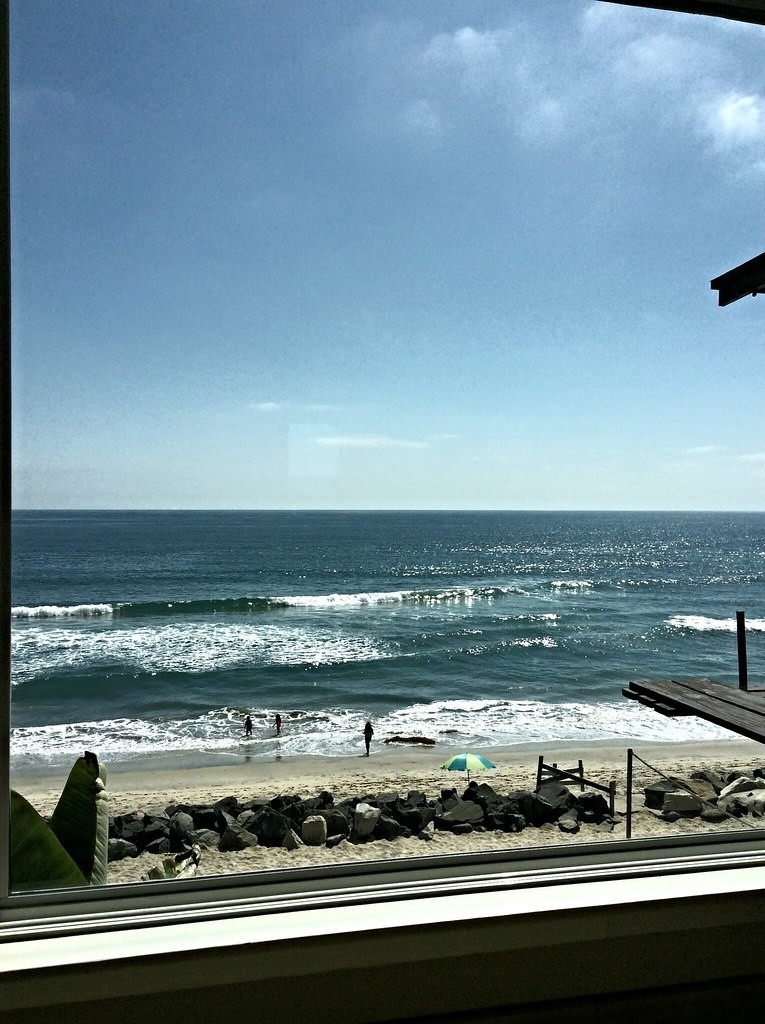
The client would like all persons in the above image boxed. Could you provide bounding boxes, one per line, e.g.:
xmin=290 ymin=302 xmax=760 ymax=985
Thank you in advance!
xmin=245 ymin=716 xmax=252 ymax=736
xmin=363 ymin=722 xmax=374 ymax=757
xmin=273 ymin=714 xmax=281 ymax=735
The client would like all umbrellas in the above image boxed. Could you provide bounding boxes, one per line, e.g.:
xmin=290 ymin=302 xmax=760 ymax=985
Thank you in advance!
xmin=440 ymin=753 xmax=496 ymax=787
